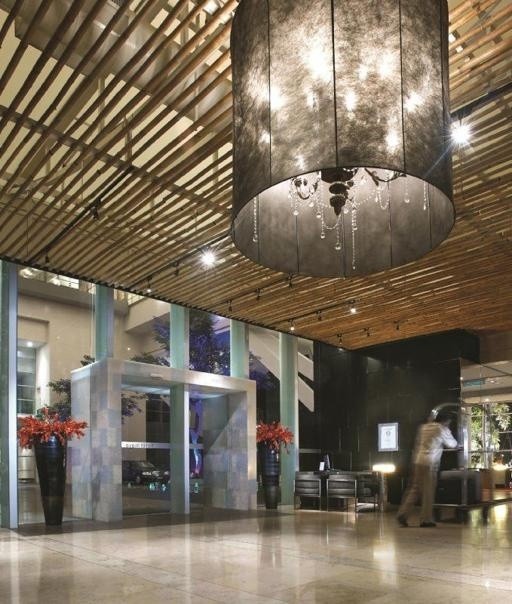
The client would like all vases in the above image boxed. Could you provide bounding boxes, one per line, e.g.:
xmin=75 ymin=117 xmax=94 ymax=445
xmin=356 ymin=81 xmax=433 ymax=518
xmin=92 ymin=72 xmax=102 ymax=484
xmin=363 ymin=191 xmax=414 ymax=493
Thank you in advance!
xmin=260 ymin=440 xmax=279 ymax=510
xmin=39 ymin=434 xmax=66 ymax=525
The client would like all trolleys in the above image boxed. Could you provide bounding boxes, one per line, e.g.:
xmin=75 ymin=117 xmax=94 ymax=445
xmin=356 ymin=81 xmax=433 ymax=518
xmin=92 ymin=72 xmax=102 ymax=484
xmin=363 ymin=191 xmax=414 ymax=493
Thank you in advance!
xmin=427 ymin=401 xmax=495 ymax=524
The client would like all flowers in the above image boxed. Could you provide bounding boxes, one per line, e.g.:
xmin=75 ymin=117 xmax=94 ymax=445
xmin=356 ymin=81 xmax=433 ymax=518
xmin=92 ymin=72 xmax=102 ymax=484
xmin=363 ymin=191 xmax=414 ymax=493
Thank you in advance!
xmin=256 ymin=421 xmax=293 ymax=456
xmin=16 ymin=407 xmax=88 ymax=449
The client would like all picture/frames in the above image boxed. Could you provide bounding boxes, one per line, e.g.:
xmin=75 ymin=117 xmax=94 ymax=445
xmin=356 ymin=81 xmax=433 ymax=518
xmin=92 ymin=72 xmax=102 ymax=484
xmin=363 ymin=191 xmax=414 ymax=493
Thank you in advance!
xmin=378 ymin=422 xmax=398 ymax=452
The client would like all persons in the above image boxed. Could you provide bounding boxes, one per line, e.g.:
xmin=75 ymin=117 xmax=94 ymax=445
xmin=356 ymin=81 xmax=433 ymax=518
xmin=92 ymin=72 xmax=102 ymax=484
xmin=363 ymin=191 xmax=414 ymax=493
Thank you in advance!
xmin=392 ymin=412 xmax=457 ymax=528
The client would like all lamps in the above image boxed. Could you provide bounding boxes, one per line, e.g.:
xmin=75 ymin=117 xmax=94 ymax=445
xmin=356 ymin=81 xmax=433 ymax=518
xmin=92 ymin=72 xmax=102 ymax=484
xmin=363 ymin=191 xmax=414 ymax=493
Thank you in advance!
xmin=227 ymin=0 xmax=456 ymax=282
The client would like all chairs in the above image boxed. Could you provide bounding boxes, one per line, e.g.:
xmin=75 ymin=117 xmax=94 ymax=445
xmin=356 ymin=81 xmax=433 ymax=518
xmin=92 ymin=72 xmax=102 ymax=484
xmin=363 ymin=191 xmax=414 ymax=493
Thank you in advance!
xmin=294 ymin=469 xmax=377 ymax=513
